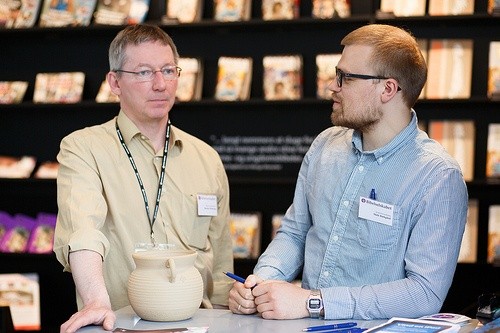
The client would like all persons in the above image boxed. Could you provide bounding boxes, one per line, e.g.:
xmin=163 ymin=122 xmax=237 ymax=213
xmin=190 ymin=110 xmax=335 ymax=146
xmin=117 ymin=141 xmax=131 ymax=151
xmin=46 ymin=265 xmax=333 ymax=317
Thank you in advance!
xmin=53 ymin=24 xmax=234 ymax=333
xmin=229 ymin=24 xmax=468 ymax=321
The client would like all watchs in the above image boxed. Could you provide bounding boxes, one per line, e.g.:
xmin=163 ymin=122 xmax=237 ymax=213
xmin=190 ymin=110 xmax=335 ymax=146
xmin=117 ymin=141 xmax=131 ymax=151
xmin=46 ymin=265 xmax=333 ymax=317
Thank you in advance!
xmin=306 ymin=289 xmax=323 ymax=319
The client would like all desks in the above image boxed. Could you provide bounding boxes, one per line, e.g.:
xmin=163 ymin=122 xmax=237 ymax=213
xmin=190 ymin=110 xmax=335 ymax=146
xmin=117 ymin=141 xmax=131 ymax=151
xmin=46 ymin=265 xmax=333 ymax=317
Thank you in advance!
xmin=78 ymin=309 xmax=480 ymax=333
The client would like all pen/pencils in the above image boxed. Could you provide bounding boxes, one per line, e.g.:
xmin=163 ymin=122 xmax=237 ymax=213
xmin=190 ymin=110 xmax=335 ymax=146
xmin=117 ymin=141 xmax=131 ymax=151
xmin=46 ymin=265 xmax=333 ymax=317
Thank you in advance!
xmin=321 ymin=327 xmax=368 ymax=333
xmin=302 ymin=322 xmax=357 ymax=331
xmin=370 ymin=189 xmax=375 ymax=199
xmin=222 ymin=271 xmax=256 ymax=288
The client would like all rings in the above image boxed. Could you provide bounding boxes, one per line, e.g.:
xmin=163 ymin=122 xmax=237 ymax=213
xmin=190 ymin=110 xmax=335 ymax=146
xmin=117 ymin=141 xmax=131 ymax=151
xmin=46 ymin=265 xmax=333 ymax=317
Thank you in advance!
xmin=237 ymin=305 xmax=241 ymax=311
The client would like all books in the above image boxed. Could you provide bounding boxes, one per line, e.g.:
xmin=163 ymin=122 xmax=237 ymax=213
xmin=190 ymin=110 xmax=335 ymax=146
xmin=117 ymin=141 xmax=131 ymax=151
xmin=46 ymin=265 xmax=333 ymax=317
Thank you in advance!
xmin=0 ymin=0 xmax=349 ymax=28
xmin=175 ymin=54 xmax=340 ymax=101
xmin=0 ymin=157 xmax=58 ymax=178
xmin=0 ymin=213 xmax=56 ymax=254
xmin=418 ymin=38 xmax=500 ymax=100
xmin=0 ymin=73 xmax=120 ymax=104
xmin=458 ymin=200 xmax=500 ymax=262
xmin=380 ymin=0 xmax=500 ymax=17
xmin=419 ymin=121 xmax=500 ymax=180
xmin=0 ymin=274 xmax=41 ymax=332
xmin=232 ymin=214 xmax=285 ymax=258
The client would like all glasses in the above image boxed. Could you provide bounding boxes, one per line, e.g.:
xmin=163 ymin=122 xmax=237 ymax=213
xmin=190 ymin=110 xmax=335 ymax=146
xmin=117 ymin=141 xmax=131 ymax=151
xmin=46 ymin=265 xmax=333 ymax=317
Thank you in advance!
xmin=113 ymin=66 xmax=182 ymax=82
xmin=336 ymin=65 xmax=401 ymax=92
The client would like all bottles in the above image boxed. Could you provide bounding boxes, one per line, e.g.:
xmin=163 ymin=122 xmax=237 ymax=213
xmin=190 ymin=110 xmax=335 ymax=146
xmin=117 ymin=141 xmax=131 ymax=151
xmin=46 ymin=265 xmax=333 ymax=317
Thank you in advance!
xmin=127 ymin=249 xmax=204 ymax=322
